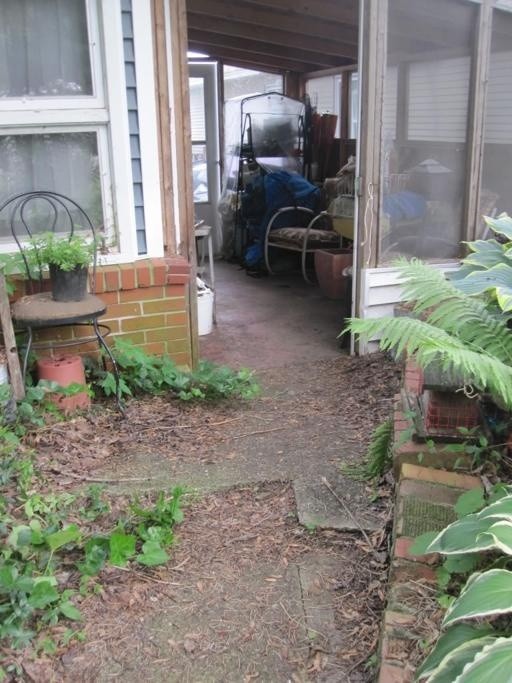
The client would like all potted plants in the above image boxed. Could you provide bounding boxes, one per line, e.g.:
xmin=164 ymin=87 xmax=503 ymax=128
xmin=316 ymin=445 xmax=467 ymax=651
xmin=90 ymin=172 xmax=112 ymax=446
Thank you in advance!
xmin=1 ymin=230 xmax=97 ymax=302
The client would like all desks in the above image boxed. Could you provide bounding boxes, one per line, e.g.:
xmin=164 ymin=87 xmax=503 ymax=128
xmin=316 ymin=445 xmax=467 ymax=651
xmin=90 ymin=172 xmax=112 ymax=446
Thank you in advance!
xmin=193 ymin=221 xmax=216 ymax=298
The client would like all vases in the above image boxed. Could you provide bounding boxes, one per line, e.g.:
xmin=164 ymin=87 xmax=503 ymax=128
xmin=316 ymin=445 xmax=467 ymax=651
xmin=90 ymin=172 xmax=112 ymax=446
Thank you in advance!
xmin=35 ymin=352 xmax=87 ymax=412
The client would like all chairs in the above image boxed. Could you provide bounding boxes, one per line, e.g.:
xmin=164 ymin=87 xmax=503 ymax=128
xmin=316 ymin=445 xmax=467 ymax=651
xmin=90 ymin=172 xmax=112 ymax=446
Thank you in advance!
xmin=263 ymin=168 xmax=370 ymax=285
xmin=0 ymin=189 xmax=119 ymax=412
